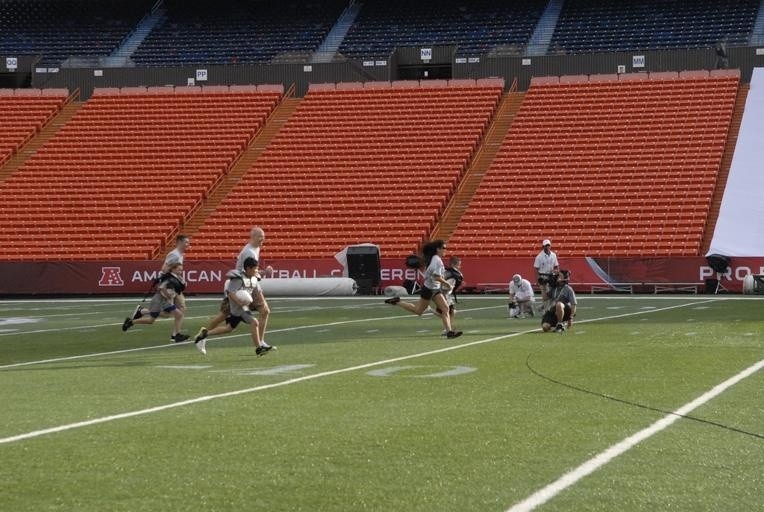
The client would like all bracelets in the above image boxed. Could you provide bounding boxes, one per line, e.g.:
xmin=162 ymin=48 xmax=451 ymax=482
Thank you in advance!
xmin=569 ymin=314 xmax=573 ymax=320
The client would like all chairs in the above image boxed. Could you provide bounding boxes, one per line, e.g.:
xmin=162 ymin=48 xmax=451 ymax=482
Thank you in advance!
xmin=0 ymin=1 xmax=763 ymax=262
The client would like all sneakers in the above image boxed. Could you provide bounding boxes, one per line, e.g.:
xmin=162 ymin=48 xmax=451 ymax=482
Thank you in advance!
xmin=194 ymin=327 xmax=209 ymax=344
xmin=175 ymin=333 xmax=191 ymax=343
xmin=536 ymin=304 xmax=545 ymax=317
xmin=169 ymin=335 xmax=175 ymax=342
xmin=552 ymin=324 xmax=565 ymax=333
xmin=440 ymin=326 xmax=456 ymax=336
xmin=448 ymin=331 xmax=463 ymax=339
xmin=515 ymin=312 xmax=525 ymax=319
xmin=262 ymin=342 xmax=276 ymax=351
xmin=385 ymin=296 xmax=400 ymax=305
xmin=122 ymin=317 xmax=134 ymax=332
xmin=196 ymin=339 xmax=206 ymax=356
xmin=256 ymin=346 xmax=272 ymax=355
xmin=422 ymin=305 xmax=432 ymax=314
xmin=527 ymin=305 xmax=535 ymax=318
xmin=132 ymin=305 xmax=144 ymax=319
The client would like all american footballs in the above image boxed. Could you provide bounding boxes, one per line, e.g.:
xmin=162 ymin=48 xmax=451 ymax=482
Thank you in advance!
xmin=160 ymin=288 xmax=177 ymax=299
xmin=442 ymin=278 xmax=456 ymax=292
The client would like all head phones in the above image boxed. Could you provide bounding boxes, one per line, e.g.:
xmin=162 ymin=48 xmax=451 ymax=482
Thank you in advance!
xmin=564 ymin=271 xmax=569 ymax=279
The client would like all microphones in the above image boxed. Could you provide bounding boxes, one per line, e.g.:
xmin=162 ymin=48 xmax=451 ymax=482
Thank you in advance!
xmin=559 ymin=278 xmax=570 ymax=281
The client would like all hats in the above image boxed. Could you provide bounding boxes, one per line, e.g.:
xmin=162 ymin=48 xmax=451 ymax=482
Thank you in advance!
xmin=543 ymin=239 xmax=551 ymax=247
xmin=513 ymin=274 xmax=522 ymax=286
xmin=160 ymin=289 xmax=175 ymax=298
xmin=440 ymin=278 xmax=455 ymax=295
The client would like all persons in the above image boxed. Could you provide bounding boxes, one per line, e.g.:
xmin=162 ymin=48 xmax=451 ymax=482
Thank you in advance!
xmin=129 ymin=235 xmax=190 ymax=339
xmin=541 ymin=268 xmax=576 ymax=332
xmin=192 ymin=226 xmax=279 ymax=355
xmin=192 ymin=257 xmax=272 ymax=356
xmin=423 ymin=254 xmax=464 ymax=337
xmin=121 ymin=262 xmax=191 ymax=343
xmin=533 ymin=238 xmax=558 ymax=314
xmin=507 ymin=274 xmax=535 ymax=319
xmin=383 ymin=239 xmax=462 ymax=338
